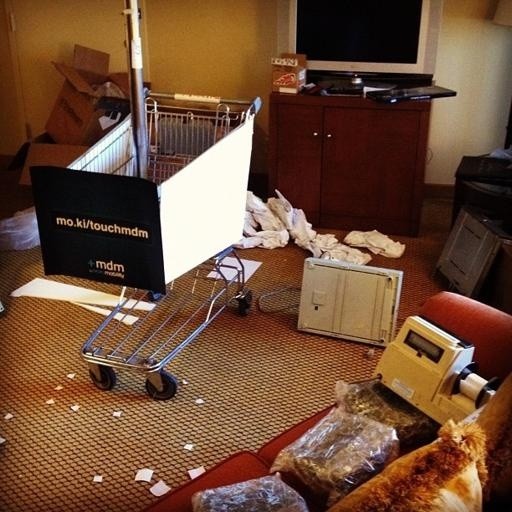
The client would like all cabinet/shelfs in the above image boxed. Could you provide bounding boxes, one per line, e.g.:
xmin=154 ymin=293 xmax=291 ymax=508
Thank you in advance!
xmin=267 ymin=84 xmax=433 ymax=241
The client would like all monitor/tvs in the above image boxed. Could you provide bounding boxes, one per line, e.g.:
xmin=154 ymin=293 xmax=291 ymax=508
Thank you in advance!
xmin=277 ymin=0 xmax=442 ymax=87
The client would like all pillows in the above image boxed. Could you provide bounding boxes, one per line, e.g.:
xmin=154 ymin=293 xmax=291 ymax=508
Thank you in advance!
xmin=328 ymin=424 xmax=502 ymax=509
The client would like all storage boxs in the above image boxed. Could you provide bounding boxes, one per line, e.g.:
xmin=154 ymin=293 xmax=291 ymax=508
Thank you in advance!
xmin=37 ymin=45 xmax=143 ymax=144
xmin=19 ymin=135 xmax=87 ymax=186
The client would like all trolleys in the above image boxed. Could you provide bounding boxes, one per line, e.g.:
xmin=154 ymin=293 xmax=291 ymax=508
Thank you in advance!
xmin=28 ymin=85 xmax=264 ymax=399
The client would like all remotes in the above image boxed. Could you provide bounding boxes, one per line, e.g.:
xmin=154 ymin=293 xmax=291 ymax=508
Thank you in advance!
xmin=327 ymin=84 xmax=363 ymax=94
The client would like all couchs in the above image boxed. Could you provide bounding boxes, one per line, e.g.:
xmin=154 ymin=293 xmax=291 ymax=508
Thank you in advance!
xmin=143 ymin=291 xmax=511 ymax=509
